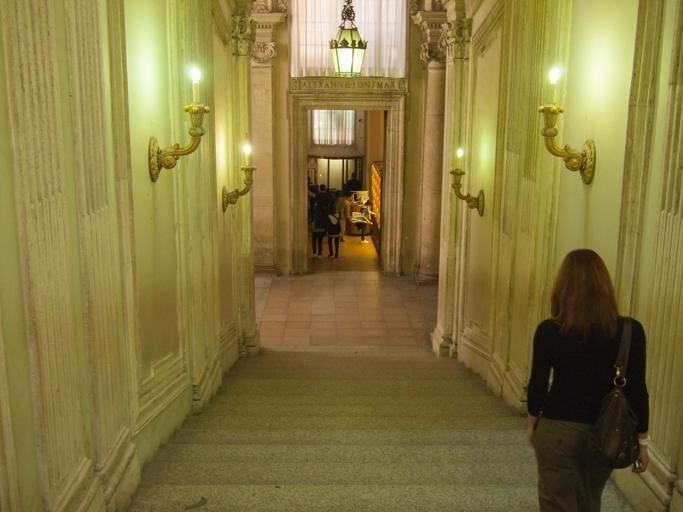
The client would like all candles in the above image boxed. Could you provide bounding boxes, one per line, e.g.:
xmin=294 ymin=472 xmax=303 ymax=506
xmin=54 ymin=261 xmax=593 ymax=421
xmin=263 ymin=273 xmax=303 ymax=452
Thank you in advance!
xmin=190 ymin=67 xmax=201 ymax=105
xmin=548 ymin=65 xmax=560 ymax=103
xmin=243 ymin=145 xmax=253 ymax=166
xmin=456 ymin=148 xmax=463 ymax=169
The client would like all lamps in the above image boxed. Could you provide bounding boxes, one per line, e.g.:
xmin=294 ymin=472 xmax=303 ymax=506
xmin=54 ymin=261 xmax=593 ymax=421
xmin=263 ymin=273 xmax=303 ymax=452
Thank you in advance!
xmin=328 ymin=0 xmax=368 ymax=78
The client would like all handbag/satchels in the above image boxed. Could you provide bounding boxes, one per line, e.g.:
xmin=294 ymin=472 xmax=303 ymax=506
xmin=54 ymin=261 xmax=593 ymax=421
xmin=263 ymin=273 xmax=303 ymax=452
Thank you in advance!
xmin=593 ymin=387 xmax=641 ymax=470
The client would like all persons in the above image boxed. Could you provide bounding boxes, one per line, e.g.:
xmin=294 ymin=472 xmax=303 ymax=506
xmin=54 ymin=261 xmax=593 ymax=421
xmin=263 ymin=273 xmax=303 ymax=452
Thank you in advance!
xmin=359 ymin=200 xmax=375 ymax=243
xmin=525 ymin=248 xmax=651 ymax=512
xmin=345 ymin=172 xmax=362 ymax=190
xmin=307 ymin=184 xmax=354 ymax=259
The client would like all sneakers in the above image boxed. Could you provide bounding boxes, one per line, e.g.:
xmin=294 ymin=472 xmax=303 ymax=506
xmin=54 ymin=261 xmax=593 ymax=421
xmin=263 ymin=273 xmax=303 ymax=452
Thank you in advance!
xmin=360 ymin=239 xmax=369 ymax=244
xmin=311 ymin=253 xmax=338 ymax=259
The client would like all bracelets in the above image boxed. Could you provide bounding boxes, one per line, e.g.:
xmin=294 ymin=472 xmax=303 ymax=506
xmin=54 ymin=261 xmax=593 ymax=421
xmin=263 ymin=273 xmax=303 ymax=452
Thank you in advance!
xmin=638 ymin=435 xmax=651 ymax=445
